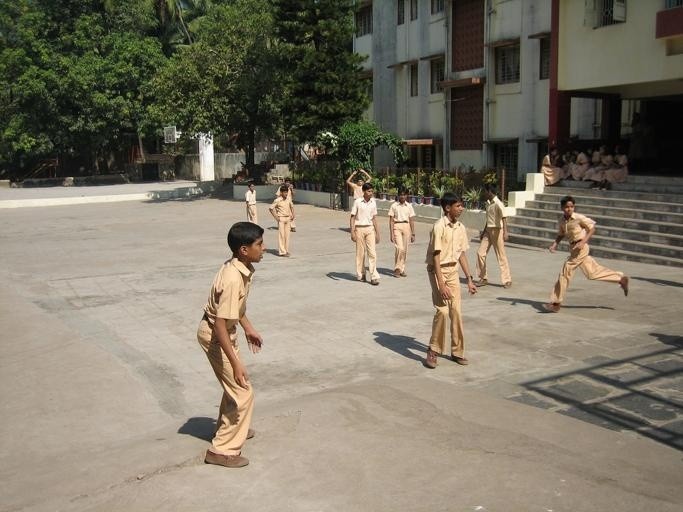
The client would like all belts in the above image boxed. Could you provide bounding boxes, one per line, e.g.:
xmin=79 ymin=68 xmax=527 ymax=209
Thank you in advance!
xmin=441 ymin=262 xmax=456 ymax=267
xmin=395 ymin=221 xmax=408 ymax=223
xmin=569 ymin=239 xmax=583 ymax=245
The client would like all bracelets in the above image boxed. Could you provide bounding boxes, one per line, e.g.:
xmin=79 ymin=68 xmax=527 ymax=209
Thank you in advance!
xmin=466 ymin=275 xmax=473 ymax=281
xmin=412 ymin=232 xmax=416 ymax=236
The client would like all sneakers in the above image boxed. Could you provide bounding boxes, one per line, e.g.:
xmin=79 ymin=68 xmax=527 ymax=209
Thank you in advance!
xmin=212 ymin=427 xmax=254 ymax=440
xmin=543 ymin=303 xmax=561 ymax=313
xmin=279 ymin=252 xmax=290 ymax=257
xmin=291 ymin=227 xmax=296 ymax=232
xmin=591 ymin=180 xmax=611 ymax=190
xmin=427 ymin=348 xmax=438 ymax=368
xmin=475 ymin=278 xmax=487 ymax=287
xmin=360 ymin=275 xmax=379 ymax=285
xmin=451 ymin=353 xmax=468 ymax=365
xmin=394 ymin=268 xmax=407 ymax=277
xmin=503 ymin=282 xmax=512 ymax=288
xmin=619 ymin=277 xmax=628 ymax=296
xmin=206 ymin=448 xmax=249 ymax=468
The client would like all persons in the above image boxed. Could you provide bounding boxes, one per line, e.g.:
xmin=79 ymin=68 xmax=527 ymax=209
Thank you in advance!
xmin=542 ymin=111 xmax=656 ymax=192
xmin=269 ymin=183 xmax=295 ymax=257
xmin=540 ymin=195 xmax=629 ymax=313
xmin=244 ymin=183 xmax=258 ymax=224
xmin=275 ymin=181 xmax=297 ymax=232
xmin=424 ymin=191 xmax=479 ymax=369
xmin=387 ymin=185 xmax=416 ymax=278
xmin=196 ymin=221 xmax=266 ymax=468
xmin=472 ymin=181 xmax=512 ymax=289
xmin=348 ymin=183 xmax=381 ymax=286
xmin=347 ymin=168 xmax=372 ymax=204
